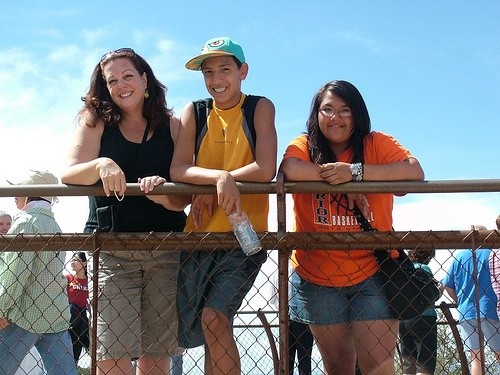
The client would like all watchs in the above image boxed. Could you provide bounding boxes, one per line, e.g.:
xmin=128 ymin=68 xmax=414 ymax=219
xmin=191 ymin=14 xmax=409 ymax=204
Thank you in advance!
xmin=350 ymin=164 xmax=358 ymax=180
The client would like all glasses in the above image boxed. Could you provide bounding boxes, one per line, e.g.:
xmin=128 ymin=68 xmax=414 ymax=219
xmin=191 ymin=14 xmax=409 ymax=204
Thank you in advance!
xmin=101 ymin=48 xmax=136 ymax=61
xmin=321 ymin=106 xmax=353 ymax=117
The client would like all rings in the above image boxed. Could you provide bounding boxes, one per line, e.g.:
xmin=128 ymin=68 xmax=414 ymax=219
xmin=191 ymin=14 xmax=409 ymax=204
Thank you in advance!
xmin=332 ymin=169 xmax=334 ymax=174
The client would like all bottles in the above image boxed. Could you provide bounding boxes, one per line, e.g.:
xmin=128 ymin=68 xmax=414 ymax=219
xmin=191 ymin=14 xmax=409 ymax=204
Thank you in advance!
xmin=227 ymin=204 xmax=262 ymax=256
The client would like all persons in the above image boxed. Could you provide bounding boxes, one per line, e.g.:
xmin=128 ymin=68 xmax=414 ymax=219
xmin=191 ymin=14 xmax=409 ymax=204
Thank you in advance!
xmin=279 ymin=80 xmax=425 ymax=375
xmin=169 ymin=37 xmax=278 ymax=375
xmin=445 ymin=224 xmax=500 ymax=375
xmin=0 ymin=170 xmax=90 ymax=375
xmin=60 ymin=48 xmax=192 ymax=375
xmin=489 ymin=215 xmax=500 ymax=318
xmin=398 ymin=248 xmax=445 ymax=375
xmin=270 ymin=249 xmax=314 ymax=375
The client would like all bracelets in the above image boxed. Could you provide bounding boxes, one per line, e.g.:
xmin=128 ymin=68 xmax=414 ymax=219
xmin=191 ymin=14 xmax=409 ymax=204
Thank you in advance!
xmin=356 ymin=163 xmax=362 ymax=182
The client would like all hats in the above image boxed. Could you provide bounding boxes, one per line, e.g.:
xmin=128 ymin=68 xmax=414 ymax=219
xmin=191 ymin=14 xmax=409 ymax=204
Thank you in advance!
xmin=8 ymin=169 xmax=58 ymax=204
xmin=185 ymin=37 xmax=246 ymax=70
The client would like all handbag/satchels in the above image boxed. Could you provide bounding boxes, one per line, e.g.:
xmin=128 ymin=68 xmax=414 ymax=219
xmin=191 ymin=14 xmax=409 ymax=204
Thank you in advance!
xmin=389 ymin=259 xmax=443 ymax=320
